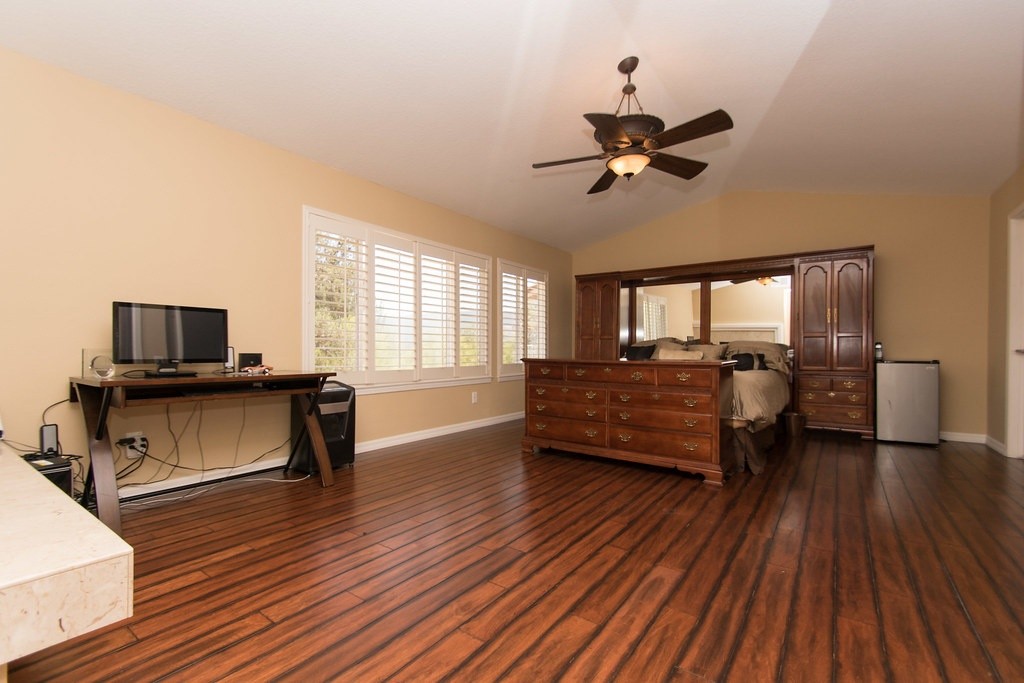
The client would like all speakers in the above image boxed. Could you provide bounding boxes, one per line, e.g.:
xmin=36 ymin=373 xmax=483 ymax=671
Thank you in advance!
xmin=238 ymin=353 xmax=263 ymax=373
xmin=290 ymin=380 xmax=356 ymax=474
xmin=222 ymin=347 xmax=236 ymax=374
xmin=39 ymin=424 xmax=58 ymax=458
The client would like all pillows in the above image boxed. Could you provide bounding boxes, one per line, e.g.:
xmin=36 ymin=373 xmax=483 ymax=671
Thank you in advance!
xmin=651 ymin=340 xmax=790 ymax=376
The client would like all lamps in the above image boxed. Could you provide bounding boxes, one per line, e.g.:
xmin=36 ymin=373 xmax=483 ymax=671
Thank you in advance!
xmin=606 ymin=153 xmax=650 ymax=180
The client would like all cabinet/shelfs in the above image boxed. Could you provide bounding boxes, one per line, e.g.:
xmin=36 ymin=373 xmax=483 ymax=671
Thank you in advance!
xmin=574 ymin=272 xmax=620 ymax=359
xmin=795 ymin=246 xmax=875 ymax=443
xmin=522 ymin=358 xmax=736 ymax=487
xmin=69 ymin=370 xmax=336 ymax=540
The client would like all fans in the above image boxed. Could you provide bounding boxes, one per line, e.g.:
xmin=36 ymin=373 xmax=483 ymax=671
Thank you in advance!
xmin=532 ymin=56 xmax=735 ymax=194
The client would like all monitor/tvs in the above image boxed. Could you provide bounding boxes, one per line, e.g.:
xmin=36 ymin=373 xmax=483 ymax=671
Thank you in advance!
xmin=112 ymin=301 xmax=228 ymax=377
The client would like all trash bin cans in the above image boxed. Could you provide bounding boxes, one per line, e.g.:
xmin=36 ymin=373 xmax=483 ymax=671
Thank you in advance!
xmin=783 ymin=412 xmax=799 ymax=438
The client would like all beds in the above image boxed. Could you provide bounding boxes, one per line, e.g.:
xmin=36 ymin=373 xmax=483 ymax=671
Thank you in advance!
xmin=624 ymin=340 xmax=793 ymax=469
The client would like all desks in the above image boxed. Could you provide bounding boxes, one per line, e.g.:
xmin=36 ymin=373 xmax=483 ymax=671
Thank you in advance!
xmin=0 ymin=440 xmax=133 ymax=665
